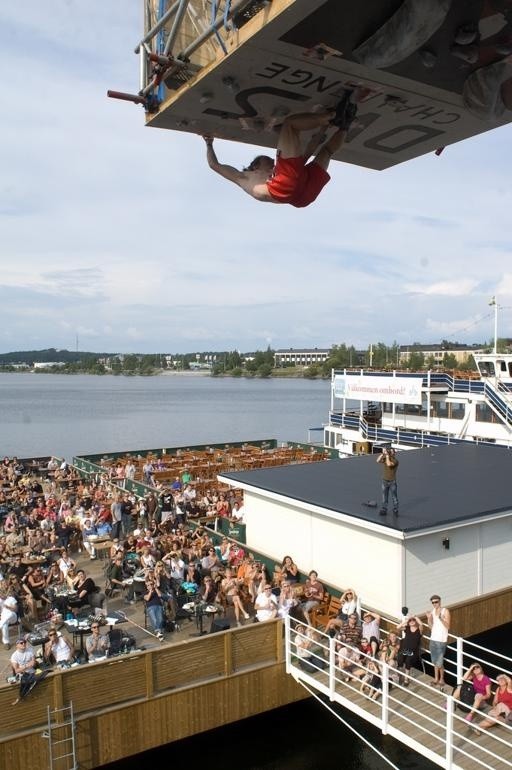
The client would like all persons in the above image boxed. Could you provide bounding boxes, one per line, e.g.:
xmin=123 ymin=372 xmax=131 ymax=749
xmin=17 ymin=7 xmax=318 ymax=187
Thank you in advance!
xmin=468 ymin=673 xmax=512 ymax=736
xmin=202 ymin=99 xmax=358 ymax=210
xmin=2 ymin=457 xmax=275 ymax=683
xmin=425 ymin=594 xmax=452 ymax=687
xmin=442 ymin=662 xmax=492 ymax=723
xmin=275 ymin=554 xmax=426 ymax=701
xmin=375 ymin=446 xmax=400 ymax=516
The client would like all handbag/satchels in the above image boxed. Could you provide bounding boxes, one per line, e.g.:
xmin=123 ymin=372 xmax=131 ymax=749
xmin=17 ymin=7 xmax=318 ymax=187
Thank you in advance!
xmin=163 ymin=615 xmax=175 ymax=632
xmin=456 ymin=684 xmax=475 ymax=713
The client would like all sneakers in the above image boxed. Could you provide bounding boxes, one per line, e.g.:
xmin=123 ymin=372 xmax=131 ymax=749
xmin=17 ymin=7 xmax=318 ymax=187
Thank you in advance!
xmin=4 ymin=643 xmax=10 ymax=650
xmin=156 ymin=631 xmax=165 ymax=642
xmin=379 ymin=509 xmax=386 ymax=515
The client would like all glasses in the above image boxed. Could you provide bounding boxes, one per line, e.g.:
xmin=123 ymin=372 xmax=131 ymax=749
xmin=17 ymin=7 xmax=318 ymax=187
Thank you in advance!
xmin=49 ymin=633 xmax=56 ymax=636
xmin=20 ymin=641 xmax=26 ymax=644
xmin=431 ymin=601 xmax=438 ymax=604
xmin=92 ymin=626 xmax=99 ymax=628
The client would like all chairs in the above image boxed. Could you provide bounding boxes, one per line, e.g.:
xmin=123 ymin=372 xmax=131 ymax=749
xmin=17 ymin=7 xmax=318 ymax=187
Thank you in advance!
xmin=106 ymin=441 xmax=331 ymax=490
xmin=401 ymin=368 xmax=490 ymax=380
xmin=0 ymin=457 xmax=276 ymax=686
